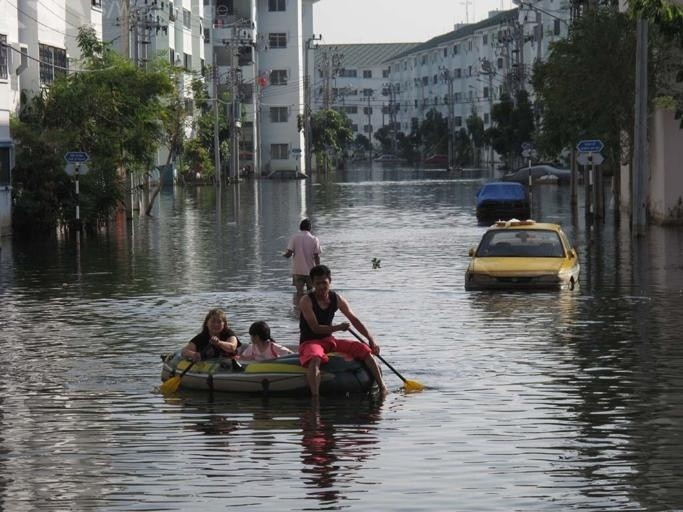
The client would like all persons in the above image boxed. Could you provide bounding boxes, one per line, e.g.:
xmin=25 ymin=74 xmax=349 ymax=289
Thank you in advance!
xmin=296 ymin=264 xmax=390 ymax=396
xmin=188 ymin=410 xmax=238 ymax=478
xmin=283 ymin=218 xmax=322 ymax=295
xmin=180 ymin=308 xmax=243 ymax=361
xmin=234 ymin=321 xmax=294 ymax=362
xmin=298 ymin=395 xmax=388 ymax=511
xmin=286 ymin=294 xmax=304 ymax=322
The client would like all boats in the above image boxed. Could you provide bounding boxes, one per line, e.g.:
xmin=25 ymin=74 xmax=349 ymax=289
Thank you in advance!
xmin=161 ymin=344 xmax=382 ymax=394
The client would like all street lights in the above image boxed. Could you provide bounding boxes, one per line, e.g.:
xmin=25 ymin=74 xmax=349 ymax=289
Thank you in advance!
xmin=511 ymin=0 xmax=568 ymax=26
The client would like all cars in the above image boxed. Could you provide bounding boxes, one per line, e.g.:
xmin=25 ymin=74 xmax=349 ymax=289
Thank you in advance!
xmin=430 ymin=154 xmax=448 ymax=163
xmin=466 ymin=165 xmax=580 ymax=290
xmin=373 ymin=154 xmax=409 ymax=162
xmin=266 ymin=170 xmax=309 ymax=179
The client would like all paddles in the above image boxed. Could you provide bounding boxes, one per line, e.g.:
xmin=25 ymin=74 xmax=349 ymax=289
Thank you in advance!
xmin=160 ymin=342 xmax=214 ymax=394
xmin=347 ymin=328 xmax=423 ymax=390
